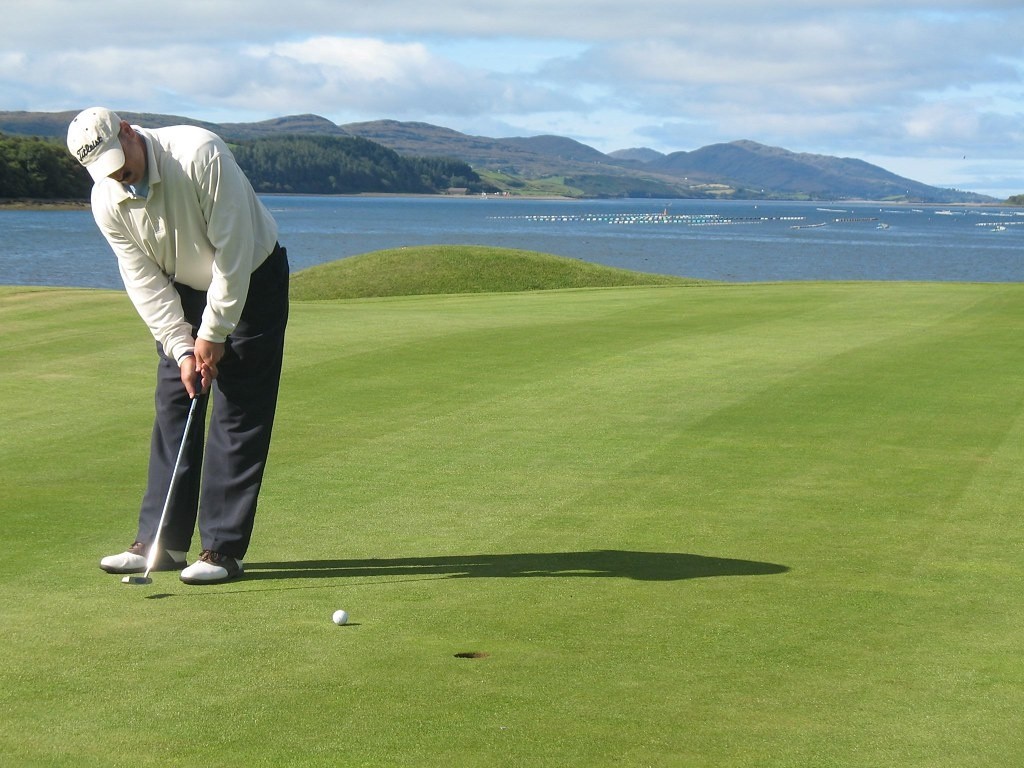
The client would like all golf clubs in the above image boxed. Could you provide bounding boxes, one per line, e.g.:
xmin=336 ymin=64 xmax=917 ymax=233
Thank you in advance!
xmin=121 ymin=372 xmax=203 ymax=585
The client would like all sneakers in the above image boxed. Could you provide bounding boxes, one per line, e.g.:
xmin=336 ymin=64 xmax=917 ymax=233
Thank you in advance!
xmin=180 ymin=550 xmax=244 ymax=585
xmin=101 ymin=539 xmax=186 ymax=573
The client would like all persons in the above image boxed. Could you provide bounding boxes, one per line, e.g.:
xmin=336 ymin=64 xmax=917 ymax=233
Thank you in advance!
xmin=66 ymin=107 xmax=289 ymax=583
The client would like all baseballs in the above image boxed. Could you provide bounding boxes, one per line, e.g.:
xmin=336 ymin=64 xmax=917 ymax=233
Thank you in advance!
xmin=333 ymin=610 xmax=348 ymax=626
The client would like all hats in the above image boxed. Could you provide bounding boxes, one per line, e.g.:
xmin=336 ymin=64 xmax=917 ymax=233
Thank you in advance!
xmin=67 ymin=106 xmax=126 ymax=182
